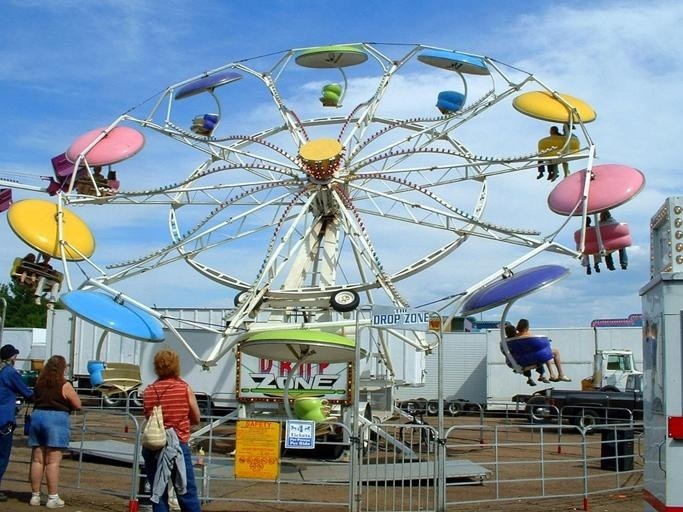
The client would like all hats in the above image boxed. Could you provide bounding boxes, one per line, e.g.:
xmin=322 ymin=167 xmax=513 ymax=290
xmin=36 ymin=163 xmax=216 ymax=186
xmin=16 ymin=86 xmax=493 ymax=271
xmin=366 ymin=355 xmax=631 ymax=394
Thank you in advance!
xmin=0 ymin=345 xmax=19 ymax=360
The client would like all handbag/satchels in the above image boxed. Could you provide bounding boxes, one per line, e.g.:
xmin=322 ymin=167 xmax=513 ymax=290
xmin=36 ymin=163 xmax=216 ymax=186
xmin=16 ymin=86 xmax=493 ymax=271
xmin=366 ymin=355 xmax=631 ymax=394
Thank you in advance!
xmin=24 ymin=415 xmax=31 ymax=435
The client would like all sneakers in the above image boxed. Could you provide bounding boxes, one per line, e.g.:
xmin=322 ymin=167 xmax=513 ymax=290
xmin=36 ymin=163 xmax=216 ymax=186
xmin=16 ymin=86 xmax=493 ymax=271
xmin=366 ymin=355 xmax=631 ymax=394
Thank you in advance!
xmin=587 ymin=263 xmax=627 ymax=273
xmin=35 ymin=297 xmax=55 ymax=311
xmin=538 ymin=375 xmax=571 ymax=383
xmin=30 ymin=495 xmax=48 ymax=505
xmin=527 ymin=380 xmax=536 ymax=386
xmin=46 ymin=498 xmax=65 ymax=508
xmin=537 ymin=173 xmax=559 ymax=182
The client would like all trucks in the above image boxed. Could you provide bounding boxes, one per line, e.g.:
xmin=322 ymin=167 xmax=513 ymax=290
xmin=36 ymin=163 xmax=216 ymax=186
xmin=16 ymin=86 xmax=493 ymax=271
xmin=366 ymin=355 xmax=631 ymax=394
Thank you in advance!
xmin=510 ymin=348 xmax=644 ymax=424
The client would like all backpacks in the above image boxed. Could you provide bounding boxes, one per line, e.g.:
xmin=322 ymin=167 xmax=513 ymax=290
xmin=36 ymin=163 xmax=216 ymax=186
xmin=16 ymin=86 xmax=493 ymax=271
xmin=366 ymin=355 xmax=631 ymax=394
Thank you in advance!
xmin=141 ymin=380 xmax=178 ymax=450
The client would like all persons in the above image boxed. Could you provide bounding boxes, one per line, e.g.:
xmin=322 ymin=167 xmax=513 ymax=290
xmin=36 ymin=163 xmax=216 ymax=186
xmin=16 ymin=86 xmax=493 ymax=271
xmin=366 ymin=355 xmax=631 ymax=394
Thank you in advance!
xmin=0 ymin=344 xmax=35 ymax=501
xmin=72 ymin=166 xmax=104 ymax=207
xmin=26 ymin=355 xmax=82 ymax=509
xmin=141 ymin=349 xmax=201 ymax=512
xmin=32 ymin=254 xmax=60 ymax=311
xmin=582 ymin=217 xmax=601 ymax=275
xmin=598 ymin=210 xmax=628 ymax=270
xmin=515 ymin=319 xmax=571 ymax=382
xmin=551 ymin=124 xmax=576 ymax=182
xmin=18 ymin=253 xmax=37 ymax=288
xmin=505 ymin=325 xmax=550 ymax=386
xmin=537 ymin=126 xmax=562 ymax=180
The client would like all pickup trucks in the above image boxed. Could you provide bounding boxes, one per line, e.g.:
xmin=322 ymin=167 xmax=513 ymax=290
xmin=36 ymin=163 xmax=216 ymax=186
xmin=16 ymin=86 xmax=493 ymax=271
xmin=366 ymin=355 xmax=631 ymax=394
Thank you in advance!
xmin=537 ymin=386 xmax=644 ymax=433
